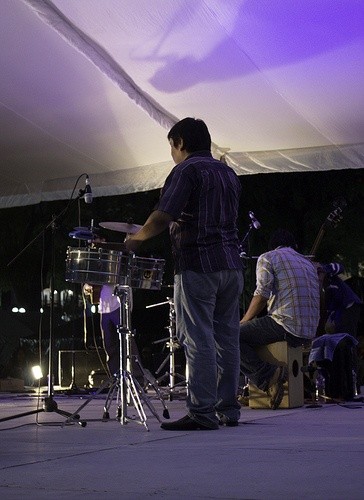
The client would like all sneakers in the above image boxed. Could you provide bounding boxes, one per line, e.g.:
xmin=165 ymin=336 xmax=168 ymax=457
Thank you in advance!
xmin=161 ymin=415 xmax=239 ymax=431
xmin=265 ymin=362 xmax=286 ymax=410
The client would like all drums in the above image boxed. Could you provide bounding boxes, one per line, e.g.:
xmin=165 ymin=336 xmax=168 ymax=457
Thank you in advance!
xmin=122 ymin=255 xmax=166 ymax=290
xmin=65 ymin=246 xmax=122 ymax=286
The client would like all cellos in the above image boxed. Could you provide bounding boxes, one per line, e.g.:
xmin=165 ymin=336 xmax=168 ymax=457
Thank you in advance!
xmin=306 ymin=199 xmax=348 ymax=333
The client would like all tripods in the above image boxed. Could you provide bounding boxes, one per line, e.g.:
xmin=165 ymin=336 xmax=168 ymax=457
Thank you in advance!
xmin=0 ymin=188 xmax=186 ymax=432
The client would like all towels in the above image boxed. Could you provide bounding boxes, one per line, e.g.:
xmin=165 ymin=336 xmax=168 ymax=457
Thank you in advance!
xmin=307 ymin=331 xmax=359 ymax=364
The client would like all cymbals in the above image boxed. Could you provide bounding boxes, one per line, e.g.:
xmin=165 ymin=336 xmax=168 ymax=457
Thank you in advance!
xmin=98 ymin=222 xmax=144 ymax=234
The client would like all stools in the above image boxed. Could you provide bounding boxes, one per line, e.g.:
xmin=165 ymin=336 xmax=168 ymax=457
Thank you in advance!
xmin=249 ymin=341 xmax=304 ymax=409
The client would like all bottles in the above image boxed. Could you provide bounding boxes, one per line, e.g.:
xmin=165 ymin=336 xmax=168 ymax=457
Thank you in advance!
xmin=315 ymin=367 xmax=326 ymax=403
xmin=353 ymin=369 xmax=358 ymax=396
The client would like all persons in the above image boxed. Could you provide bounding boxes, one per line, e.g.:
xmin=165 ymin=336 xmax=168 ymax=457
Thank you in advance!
xmin=238 ymin=226 xmax=321 ymax=410
xmin=97 ymin=283 xmax=121 ymax=377
xmin=311 ymin=262 xmax=364 ymax=340
xmin=125 ymin=116 xmax=244 ymax=431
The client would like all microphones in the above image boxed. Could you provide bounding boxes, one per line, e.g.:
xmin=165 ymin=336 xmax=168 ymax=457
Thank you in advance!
xmin=249 ymin=211 xmax=261 ymax=229
xmin=84 ymin=175 xmax=93 ymax=204
